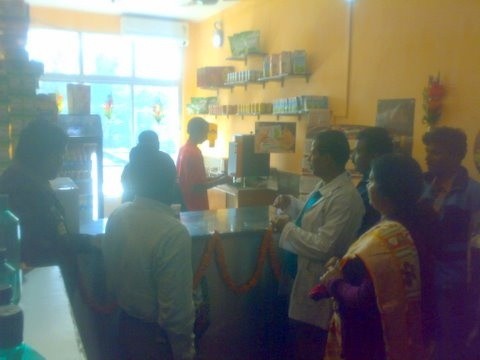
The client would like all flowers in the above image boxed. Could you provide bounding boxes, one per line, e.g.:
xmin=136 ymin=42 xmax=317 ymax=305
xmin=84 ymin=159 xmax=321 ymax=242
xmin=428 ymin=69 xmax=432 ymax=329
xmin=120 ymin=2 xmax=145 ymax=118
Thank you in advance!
xmin=422 ymin=71 xmax=445 ymax=131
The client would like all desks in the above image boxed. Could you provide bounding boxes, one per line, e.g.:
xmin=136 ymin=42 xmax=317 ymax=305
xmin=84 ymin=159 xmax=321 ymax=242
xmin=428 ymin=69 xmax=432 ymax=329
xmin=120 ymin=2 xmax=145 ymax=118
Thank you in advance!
xmin=18 ymin=265 xmax=86 ymax=359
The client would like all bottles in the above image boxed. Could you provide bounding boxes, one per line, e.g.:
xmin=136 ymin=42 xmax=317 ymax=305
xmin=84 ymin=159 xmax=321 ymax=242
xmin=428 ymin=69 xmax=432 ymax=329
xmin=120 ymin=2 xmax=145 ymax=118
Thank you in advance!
xmin=0 ymin=284 xmax=23 ymax=347
xmin=0 ymin=194 xmax=22 ymax=271
xmin=0 ymin=246 xmax=23 ymax=305
xmin=57 ymin=145 xmax=92 ymax=222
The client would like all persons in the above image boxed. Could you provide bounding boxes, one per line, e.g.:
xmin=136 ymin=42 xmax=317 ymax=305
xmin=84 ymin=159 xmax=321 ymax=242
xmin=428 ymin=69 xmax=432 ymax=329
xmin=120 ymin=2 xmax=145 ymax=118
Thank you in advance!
xmin=352 ymin=127 xmax=394 ymax=239
xmin=416 ymin=127 xmax=479 ymax=360
xmin=272 ymin=131 xmax=365 ymax=360
xmin=177 ymin=117 xmax=231 ymax=211
xmin=319 ymin=153 xmax=426 ymax=360
xmin=0 ymin=121 xmax=104 ymax=360
xmin=105 ymin=151 xmax=196 ymax=360
xmin=120 ymin=131 xmax=176 ymax=205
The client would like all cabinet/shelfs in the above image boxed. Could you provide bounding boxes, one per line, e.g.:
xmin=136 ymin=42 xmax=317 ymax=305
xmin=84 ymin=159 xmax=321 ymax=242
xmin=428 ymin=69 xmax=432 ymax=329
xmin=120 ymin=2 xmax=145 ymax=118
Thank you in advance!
xmin=207 ymin=183 xmax=268 ymax=209
xmin=188 ymin=52 xmax=332 ymax=122
